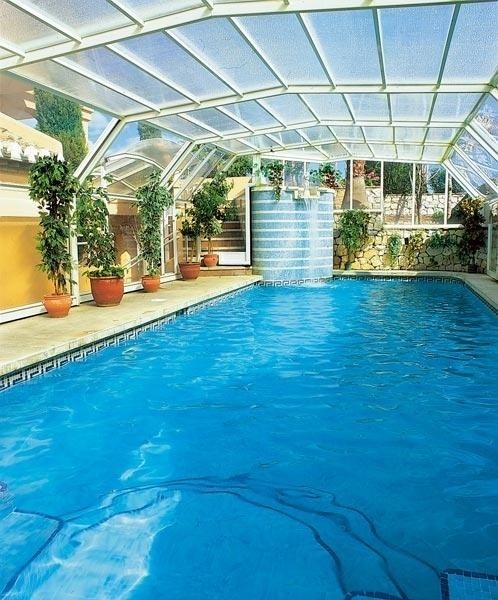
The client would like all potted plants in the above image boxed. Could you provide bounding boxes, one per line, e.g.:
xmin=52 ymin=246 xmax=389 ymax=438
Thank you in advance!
xmin=130 ymin=170 xmax=173 ymax=293
xmin=457 ymin=209 xmax=487 ymax=274
xmin=337 ymin=209 xmax=368 ymax=270
xmin=202 ymin=172 xmax=237 ymax=268
xmin=179 ymin=190 xmax=212 ymax=281
xmin=29 ymin=152 xmax=82 ymax=317
xmin=70 ymin=175 xmax=127 ymax=307
